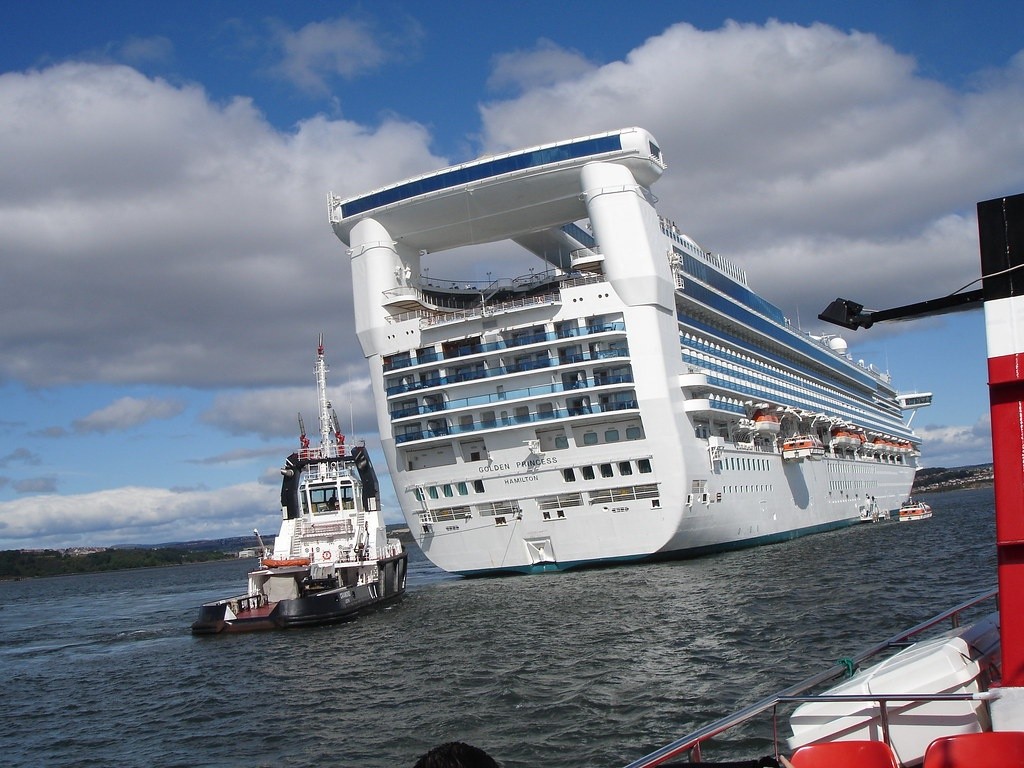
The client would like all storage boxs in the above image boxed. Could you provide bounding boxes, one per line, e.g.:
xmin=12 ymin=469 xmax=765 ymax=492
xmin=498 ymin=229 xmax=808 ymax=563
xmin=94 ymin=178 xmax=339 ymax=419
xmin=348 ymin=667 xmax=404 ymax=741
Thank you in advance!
xmin=788 ymin=617 xmax=1004 ymax=767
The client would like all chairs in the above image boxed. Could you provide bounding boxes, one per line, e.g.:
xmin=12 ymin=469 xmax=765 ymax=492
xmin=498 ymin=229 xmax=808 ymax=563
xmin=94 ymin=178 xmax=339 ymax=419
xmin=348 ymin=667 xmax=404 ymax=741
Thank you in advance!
xmin=789 ymin=729 xmax=1024 ymax=768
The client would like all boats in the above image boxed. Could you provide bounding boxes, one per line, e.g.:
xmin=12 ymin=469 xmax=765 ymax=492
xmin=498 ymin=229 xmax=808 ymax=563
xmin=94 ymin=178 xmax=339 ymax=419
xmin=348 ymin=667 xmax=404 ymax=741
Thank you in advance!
xmin=860 ymin=496 xmax=885 ymax=523
xmin=783 ymin=434 xmax=825 ymax=464
xmin=191 ymin=332 xmax=409 ymax=637
xmin=899 ymin=497 xmax=933 ymax=521
xmin=753 ymin=413 xmax=781 ymax=438
xmin=833 ymin=431 xmax=861 ymax=450
xmin=863 ymin=438 xmax=913 ymax=456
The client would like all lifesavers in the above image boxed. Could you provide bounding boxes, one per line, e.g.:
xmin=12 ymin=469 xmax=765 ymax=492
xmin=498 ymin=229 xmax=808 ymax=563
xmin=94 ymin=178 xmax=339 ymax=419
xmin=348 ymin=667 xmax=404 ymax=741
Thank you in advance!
xmin=323 ymin=551 xmax=331 ymax=560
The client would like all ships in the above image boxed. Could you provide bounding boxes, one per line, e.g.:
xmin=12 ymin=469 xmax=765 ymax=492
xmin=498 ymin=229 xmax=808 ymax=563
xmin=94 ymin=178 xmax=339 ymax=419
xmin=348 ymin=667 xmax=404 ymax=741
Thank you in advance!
xmin=326 ymin=126 xmax=932 ymax=576
